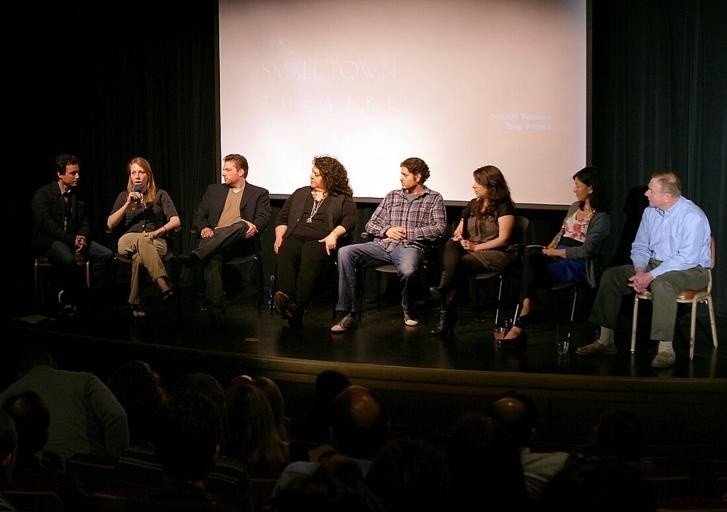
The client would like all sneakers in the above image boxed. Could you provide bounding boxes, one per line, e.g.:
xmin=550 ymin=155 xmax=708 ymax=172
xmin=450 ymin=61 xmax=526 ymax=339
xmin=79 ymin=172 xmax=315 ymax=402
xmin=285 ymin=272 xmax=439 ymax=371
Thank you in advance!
xmin=651 ymin=351 xmax=676 ymax=368
xmin=179 ymin=254 xmax=204 ymax=268
xmin=403 ymin=309 xmax=418 ymax=326
xmin=331 ymin=314 xmax=357 ymax=332
xmin=58 ymin=290 xmax=77 ymax=316
xmin=273 ymin=291 xmax=303 ymax=327
xmin=575 ymin=340 xmax=618 ymax=355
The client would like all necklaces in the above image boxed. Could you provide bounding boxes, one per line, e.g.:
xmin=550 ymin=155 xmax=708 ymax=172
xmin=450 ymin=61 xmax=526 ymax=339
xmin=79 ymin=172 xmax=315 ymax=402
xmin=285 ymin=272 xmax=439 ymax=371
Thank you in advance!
xmin=582 ymin=206 xmax=595 ymax=217
xmin=306 ymin=189 xmax=328 ymax=224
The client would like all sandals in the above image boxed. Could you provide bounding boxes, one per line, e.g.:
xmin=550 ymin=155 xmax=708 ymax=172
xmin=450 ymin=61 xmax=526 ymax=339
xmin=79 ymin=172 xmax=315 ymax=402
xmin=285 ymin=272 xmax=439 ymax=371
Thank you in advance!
xmin=161 ymin=285 xmax=174 ymax=301
xmin=131 ymin=304 xmax=146 ymax=319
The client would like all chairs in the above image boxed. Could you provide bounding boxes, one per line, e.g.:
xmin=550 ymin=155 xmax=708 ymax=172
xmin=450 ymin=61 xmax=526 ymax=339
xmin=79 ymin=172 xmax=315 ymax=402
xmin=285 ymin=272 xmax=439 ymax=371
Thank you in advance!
xmin=1 ymin=417 xmax=727 ymax=511
xmin=26 ymin=213 xmax=720 ymax=361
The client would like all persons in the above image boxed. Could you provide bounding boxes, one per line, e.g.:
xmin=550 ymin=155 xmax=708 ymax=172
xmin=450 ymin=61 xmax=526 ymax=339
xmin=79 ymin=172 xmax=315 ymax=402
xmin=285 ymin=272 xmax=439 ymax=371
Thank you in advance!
xmin=429 ymin=165 xmax=519 ymax=337
xmin=108 ymin=158 xmax=181 ymax=319
xmin=497 ymin=168 xmax=611 ymax=346
xmin=329 ymin=157 xmax=447 ymax=333
xmin=31 ymin=153 xmax=113 ymax=323
xmin=272 ymin=157 xmax=358 ymax=327
xmin=574 ymin=169 xmax=712 ymax=368
xmin=186 ymin=154 xmax=271 ymax=326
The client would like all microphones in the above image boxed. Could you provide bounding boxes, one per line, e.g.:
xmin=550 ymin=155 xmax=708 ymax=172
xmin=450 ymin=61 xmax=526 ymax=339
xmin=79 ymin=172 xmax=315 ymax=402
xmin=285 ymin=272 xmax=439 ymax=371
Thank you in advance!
xmin=134 ymin=186 xmax=142 ymax=208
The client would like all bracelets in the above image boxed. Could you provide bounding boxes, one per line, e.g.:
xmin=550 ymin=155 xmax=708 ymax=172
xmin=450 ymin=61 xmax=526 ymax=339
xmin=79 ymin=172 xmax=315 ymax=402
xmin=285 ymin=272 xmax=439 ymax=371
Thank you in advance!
xmin=163 ymin=226 xmax=168 ymax=233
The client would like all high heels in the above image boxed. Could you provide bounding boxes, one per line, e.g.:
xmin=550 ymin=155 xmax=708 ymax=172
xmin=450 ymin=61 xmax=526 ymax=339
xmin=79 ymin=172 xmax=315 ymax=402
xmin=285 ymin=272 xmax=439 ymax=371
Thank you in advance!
xmin=431 ymin=306 xmax=457 ymax=337
xmin=430 ymin=271 xmax=460 ymax=302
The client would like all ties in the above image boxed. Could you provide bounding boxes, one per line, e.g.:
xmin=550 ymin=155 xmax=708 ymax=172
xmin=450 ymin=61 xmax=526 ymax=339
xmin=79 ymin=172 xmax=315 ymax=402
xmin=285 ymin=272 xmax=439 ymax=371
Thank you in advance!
xmin=65 ymin=190 xmax=73 ymax=236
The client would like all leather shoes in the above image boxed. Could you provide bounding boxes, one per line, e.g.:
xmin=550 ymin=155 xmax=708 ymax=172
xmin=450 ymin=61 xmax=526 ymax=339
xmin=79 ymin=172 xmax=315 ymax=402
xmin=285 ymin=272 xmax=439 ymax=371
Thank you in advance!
xmin=515 ymin=308 xmax=533 ymax=328
xmin=497 ymin=333 xmax=523 ymax=349
